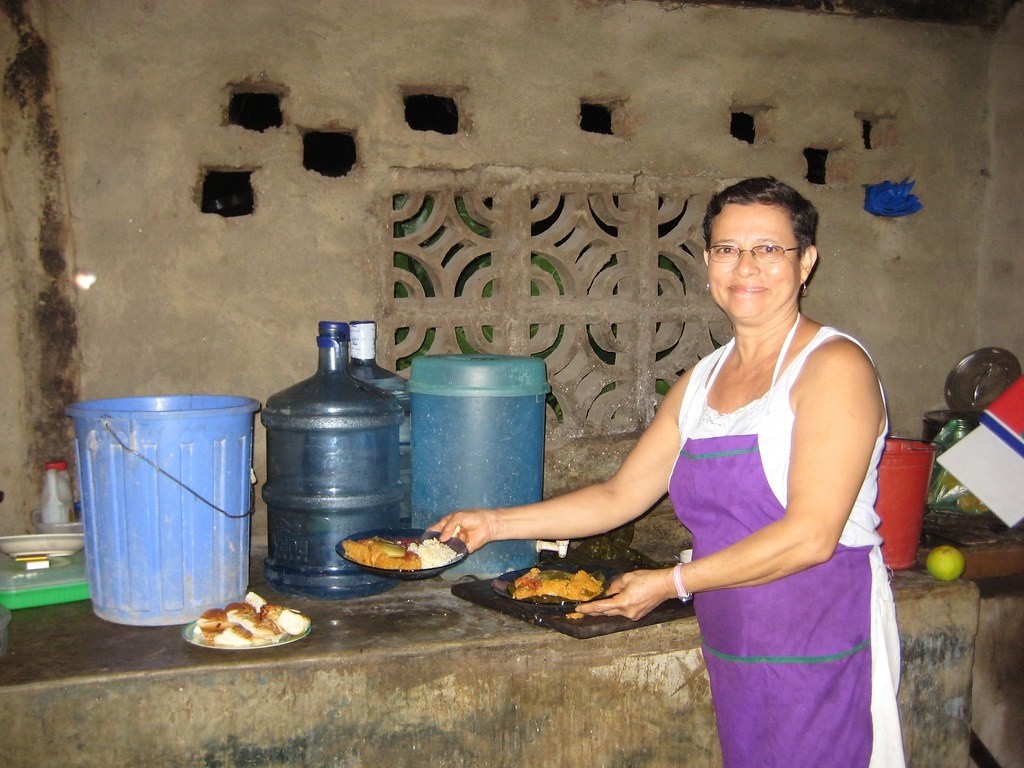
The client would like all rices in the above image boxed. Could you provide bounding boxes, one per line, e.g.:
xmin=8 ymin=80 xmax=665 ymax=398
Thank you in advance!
xmin=407 ymin=537 xmax=458 ymax=568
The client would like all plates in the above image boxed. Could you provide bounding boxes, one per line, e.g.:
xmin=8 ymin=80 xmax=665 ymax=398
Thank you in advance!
xmin=180 ymin=618 xmax=312 ymax=651
xmin=491 ymin=567 xmax=618 ymax=614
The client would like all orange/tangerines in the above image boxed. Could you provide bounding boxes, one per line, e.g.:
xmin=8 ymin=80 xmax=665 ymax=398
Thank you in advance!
xmin=927 ymin=545 xmax=964 ymax=581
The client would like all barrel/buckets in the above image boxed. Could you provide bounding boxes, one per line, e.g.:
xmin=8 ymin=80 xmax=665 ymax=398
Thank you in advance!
xmin=408 ymin=351 xmax=553 ymax=583
xmin=876 ymin=433 xmax=940 ymax=570
xmin=65 ymin=392 xmax=262 ymax=627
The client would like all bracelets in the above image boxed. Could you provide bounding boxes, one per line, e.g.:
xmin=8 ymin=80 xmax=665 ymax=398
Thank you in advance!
xmin=673 ymin=562 xmax=693 ymax=602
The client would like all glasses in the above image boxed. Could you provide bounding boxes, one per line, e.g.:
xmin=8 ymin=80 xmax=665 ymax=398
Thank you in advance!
xmin=706 ymin=244 xmax=801 ymax=264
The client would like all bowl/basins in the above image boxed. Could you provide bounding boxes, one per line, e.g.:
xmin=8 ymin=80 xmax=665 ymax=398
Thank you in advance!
xmin=336 ymin=527 xmax=470 ymax=581
xmin=0 ymin=533 xmax=86 ymax=558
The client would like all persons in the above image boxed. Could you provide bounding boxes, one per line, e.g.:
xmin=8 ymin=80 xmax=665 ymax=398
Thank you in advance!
xmin=431 ymin=175 xmax=908 ymax=768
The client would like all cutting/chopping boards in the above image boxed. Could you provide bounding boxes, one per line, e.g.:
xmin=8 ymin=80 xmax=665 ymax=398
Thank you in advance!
xmin=450 ymin=564 xmax=696 ymax=640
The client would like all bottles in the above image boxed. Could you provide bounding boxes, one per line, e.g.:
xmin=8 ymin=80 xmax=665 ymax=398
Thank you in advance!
xmin=260 ymin=320 xmax=414 ymax=600
xmin=40 ymin=460 xmax=74 ymax=525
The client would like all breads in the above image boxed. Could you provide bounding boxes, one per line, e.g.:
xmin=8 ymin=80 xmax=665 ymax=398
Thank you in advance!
xmin=341 ymin=538 xmax=422 ymax=569
xmin=194 ymin=592 xmax=311 ymax=646
